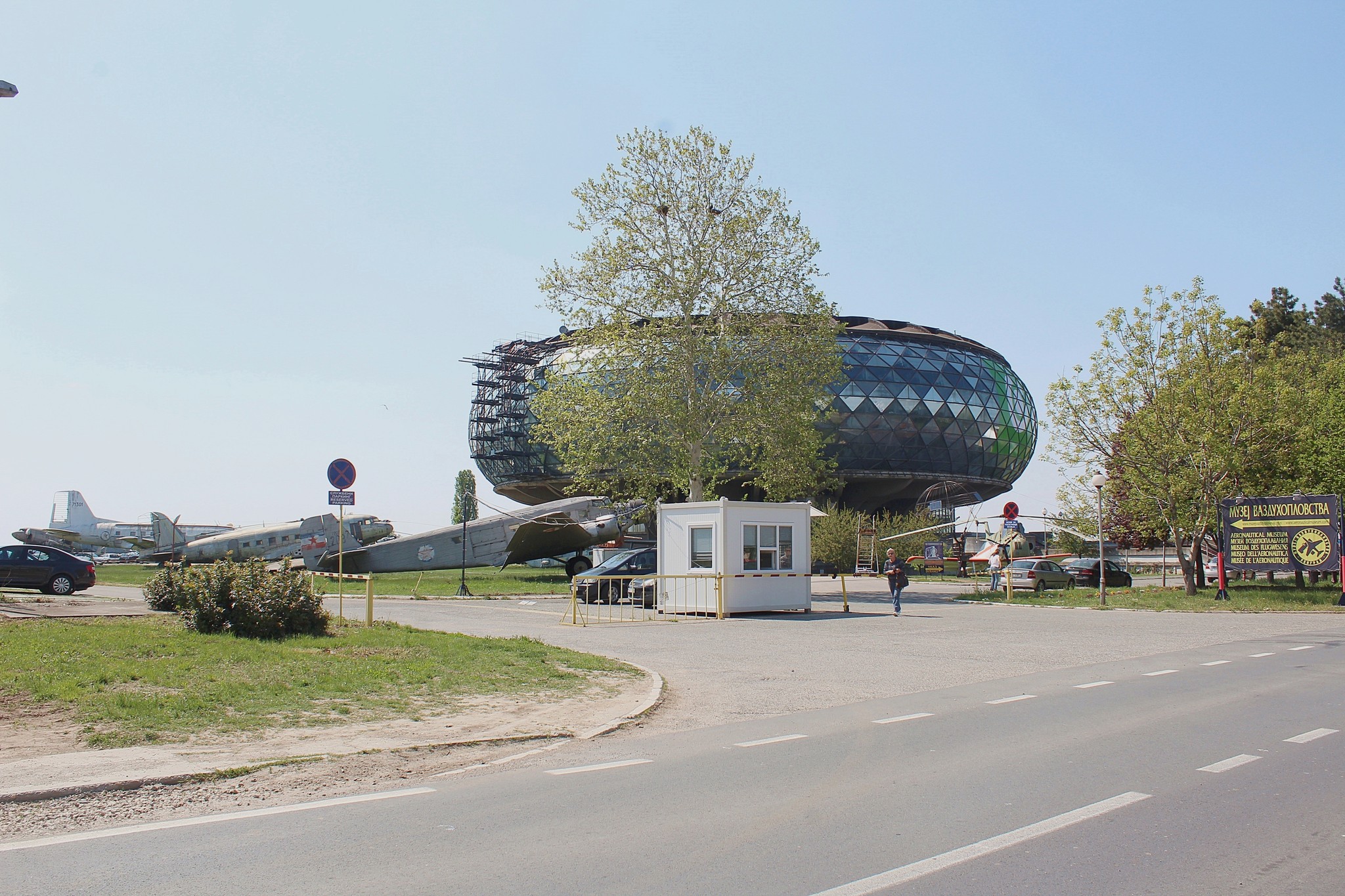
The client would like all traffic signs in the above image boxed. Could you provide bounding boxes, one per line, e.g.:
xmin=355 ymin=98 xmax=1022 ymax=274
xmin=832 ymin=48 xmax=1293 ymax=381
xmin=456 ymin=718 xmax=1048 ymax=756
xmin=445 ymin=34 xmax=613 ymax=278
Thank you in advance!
xmin=924 ymin=565 xmax=944 ymax=573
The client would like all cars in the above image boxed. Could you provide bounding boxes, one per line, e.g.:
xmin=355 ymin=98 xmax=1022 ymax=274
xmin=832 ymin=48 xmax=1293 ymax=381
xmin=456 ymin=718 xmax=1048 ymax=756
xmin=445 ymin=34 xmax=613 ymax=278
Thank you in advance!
xmin=1204 ymin=556 xmax=1256 ymax=582
xmin=1056 ymin=558 xmax=1079 ymax=567
xmin=0 ymin=545 xmax=97 ymax=596
xmin=1062 ymin=558 xmax=1132 ymax=588
xmin=74 ymin=552 xmax=145 ymax=565
xmin=627 ymin=573 xmax=658 ymax=605
xmin=1000 ymin=558 xmax=1077 ymax=593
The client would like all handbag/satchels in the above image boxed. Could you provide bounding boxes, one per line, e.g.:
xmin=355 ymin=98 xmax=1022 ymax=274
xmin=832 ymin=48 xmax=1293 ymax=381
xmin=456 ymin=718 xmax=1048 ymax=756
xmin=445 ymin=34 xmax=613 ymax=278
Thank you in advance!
xmin=895 ymin=572 xmax=909 ymax=588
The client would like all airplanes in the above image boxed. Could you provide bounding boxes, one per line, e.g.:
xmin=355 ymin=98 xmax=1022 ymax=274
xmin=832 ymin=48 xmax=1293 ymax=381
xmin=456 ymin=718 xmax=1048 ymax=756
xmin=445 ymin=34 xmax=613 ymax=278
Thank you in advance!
xmin=11 ymin=528 xmax=76 ymax=553
xmin=115 ymin=511 xmax=394 ymax=567
xmin=41 ymin=491 xmax=235 ymax=550
xmin=299 ymin=497 xmax=649 ymax=576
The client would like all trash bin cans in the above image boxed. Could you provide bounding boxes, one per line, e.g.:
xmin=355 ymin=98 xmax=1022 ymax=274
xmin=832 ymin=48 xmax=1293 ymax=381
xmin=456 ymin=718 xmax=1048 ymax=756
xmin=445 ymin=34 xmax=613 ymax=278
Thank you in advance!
xmin=820 ymin=570 xmax=824 ymax=574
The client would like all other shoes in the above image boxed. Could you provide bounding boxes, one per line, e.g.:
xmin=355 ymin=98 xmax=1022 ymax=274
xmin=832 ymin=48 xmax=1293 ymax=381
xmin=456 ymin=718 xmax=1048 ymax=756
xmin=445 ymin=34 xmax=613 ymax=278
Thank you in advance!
xmin=893 ymin=611 xmax=898 ymax=616
xmin=897 ymin=611 xmax=901 ymax=615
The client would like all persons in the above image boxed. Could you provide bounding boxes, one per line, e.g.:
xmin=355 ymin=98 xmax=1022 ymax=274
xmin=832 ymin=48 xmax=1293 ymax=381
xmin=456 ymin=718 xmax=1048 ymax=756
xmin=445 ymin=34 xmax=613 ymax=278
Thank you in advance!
xmin=883 ymin=548 xmax=906 ymax=617
xmin=637 ymin=557 xmax=646 ymax=570
xmin=780 ymin=548 xmax=791 ymax=568
xmin=988 ymin=549 xmax=1002 ymax=592
xmin=1207 ymin=560 xmax=1210 ymax=564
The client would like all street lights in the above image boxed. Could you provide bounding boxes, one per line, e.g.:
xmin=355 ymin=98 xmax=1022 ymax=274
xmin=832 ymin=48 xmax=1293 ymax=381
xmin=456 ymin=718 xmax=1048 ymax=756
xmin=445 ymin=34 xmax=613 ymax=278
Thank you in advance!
xmin=1042 ymin=507 xmax=1048 ymax=556
xmin=1092 ymin=472 xmax=1107 ymax=606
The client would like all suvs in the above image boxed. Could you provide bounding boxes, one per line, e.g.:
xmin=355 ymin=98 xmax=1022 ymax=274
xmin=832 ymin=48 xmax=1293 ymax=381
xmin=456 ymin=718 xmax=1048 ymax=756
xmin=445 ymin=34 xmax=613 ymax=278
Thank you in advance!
xmin=569 ymin=546 xmax=657 ymax=604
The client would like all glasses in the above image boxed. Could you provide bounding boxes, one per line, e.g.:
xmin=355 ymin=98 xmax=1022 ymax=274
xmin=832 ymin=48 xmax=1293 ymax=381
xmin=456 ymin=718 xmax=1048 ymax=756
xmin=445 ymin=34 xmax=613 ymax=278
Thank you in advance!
xmin=786 ymin=550 xmax=790 ymax=552
xmin=888 ymin=553 xmax=893 ymax=555
